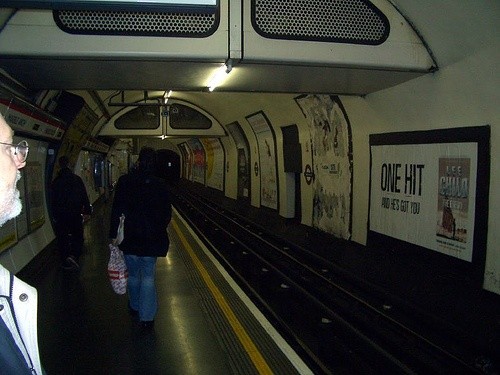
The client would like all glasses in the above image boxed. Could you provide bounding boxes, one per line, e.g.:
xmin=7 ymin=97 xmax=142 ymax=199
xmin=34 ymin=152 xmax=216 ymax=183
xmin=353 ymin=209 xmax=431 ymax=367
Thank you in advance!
xmin=0 ymin=140 xmax=30 ymax=162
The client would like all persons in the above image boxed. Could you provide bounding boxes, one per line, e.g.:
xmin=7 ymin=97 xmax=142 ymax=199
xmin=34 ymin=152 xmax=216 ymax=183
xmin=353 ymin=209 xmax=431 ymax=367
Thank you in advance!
xmin=110 ymin=147 xmax=172 ymax=327
xmin=0 ymin=113 xmax=47 ymax=375
xmin=46 ymin=155 xmax=91 ymax=271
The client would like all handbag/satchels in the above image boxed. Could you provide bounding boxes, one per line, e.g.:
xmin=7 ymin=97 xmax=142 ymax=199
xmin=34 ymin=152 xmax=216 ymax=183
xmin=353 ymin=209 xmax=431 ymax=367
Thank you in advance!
xmin=108 ymin=244 xmax=128 ymax=294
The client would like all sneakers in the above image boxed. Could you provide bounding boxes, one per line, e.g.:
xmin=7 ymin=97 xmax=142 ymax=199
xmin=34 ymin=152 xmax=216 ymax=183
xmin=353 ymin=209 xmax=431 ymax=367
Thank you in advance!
xmin=142 ymin=321 xmax=156 ymax=327
xmin=68 ymin=256 xmax=81 ymax=269
xmin=63 ymin=262 xmax=72 ymax=269
xmin=127 ymin=300 xmax=139 ymax=313
